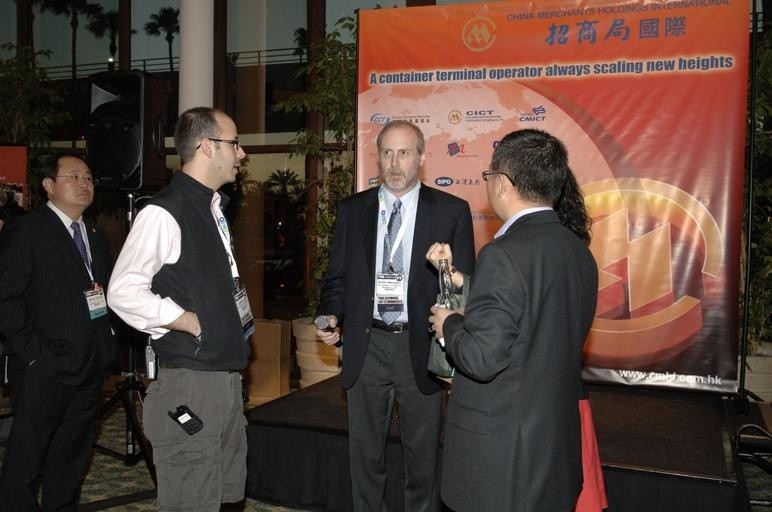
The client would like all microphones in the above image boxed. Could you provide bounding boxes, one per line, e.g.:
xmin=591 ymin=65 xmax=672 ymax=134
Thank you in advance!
xmin=314 ymin=315 xmax=343 ymax=348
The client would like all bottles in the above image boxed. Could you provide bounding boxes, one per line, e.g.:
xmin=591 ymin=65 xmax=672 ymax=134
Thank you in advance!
xmin=436 ymin=257 xmax=460 ymax=315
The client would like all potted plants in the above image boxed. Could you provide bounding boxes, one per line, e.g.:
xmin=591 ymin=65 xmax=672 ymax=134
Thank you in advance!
xmin=737 ymin=30 xmax=771 ymax=406
xmin=269 ymin=2 xmax=396 ymax=392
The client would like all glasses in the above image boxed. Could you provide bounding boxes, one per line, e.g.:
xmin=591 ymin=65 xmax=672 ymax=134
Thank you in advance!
xmin=482 ymin=171 xmax=515 ymax=187
xmin=195 ymin=137 xmax=241 ymax=152
xmin=56 ymin=175 xmax=94 ymax=184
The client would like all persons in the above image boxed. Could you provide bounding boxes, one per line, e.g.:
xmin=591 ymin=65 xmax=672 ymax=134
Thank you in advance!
xmin=429 ymin=130 xmax=599 ymax=512
xmin=0 ymin=151 xmax=135 ymax=510
xmin=316 ymin=119 xmax=476 ymax=512
xmin=0 ymin=201 xmax=23 ymax=230
xmin=108 ymin=107 xmax=253 ymax=512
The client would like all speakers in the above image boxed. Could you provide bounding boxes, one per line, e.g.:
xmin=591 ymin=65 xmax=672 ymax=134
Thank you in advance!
xmin=88 ymin=71 xmax=167 ymax=191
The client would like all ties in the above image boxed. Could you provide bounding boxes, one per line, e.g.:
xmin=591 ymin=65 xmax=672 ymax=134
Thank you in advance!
xmin=381 ymin=199 xmax=406 ymax=275
xmin=70 ymin=221 xmax=91 ymax=271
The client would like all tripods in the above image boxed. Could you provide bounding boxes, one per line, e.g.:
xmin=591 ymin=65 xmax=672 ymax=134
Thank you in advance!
xmin=92 ymin=193 xmax=158 ymax=496
